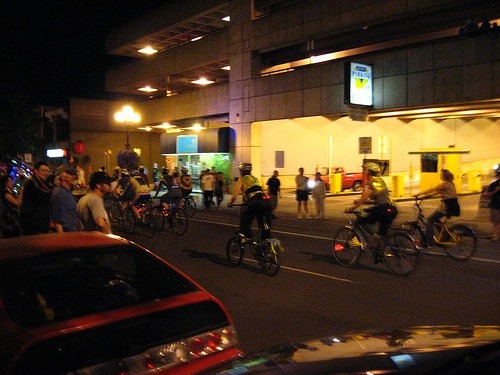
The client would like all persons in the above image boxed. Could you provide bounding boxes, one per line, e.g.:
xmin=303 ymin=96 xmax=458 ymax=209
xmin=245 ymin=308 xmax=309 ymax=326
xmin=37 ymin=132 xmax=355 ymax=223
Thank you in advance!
xmin=50 ymin=165 xmax=78 ymax=234
xmin=179 ymin=167 xmax=192 ymax=209
xmin=266 ymin=170 xmax=282 ymax=219
xmin=414 ymin=169 xmax=460 ymax=247
xmin=230 ymin=163 xmax=271 ymax=260
xmin=199 ymin=166 xmax=223 ymax=209
xmin=76 ymin=171 xmax=116 ymax=234
xmin=311 ymin=172 xmax=326 ymax=220
xmin=485 ymin=164 xmax=500 ymax=241
xmin=0 ymin=158 xmax=85 ymax=199
xmin=295 ymin=167 xmax=311 ymax=219
xmin=96 ymin=166 xmax=149 ymax=217
xmin=344 ymin=162 xmax=398 ymax=264
xmin=334 ymin=168 xmax=340 ymax=173
xmin=152 ymin=163 xmax=181 ymax=191
xmin=154 ymin=166 xmax=182 ymax=230
xmin=0 ymin=175 xmax=22 ymax=238
xmin=20 ymin=162 xmax=50 ymax=234
xmin=120 ymin=169 xmax=151 ymax=223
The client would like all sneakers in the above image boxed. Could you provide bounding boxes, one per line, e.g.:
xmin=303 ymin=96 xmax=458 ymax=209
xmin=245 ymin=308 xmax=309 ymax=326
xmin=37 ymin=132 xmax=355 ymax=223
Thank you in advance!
xmin=160 ymin=208 xmax=171 ymax=216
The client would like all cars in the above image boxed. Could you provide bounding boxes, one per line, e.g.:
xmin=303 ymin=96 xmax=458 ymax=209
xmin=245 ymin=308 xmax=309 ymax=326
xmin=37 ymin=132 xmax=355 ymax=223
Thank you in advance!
xmin=0 ymin=231 xmax=245 ymax=375
xmin=191 ymin=326 xmax=500 ymax=375
xmin=134 ymin=175 xmax=150 ymax=200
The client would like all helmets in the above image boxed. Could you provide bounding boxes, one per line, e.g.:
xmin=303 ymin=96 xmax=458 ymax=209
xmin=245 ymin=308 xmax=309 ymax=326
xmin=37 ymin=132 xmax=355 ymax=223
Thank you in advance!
xmin=181 ymin=167 xmax=188 ymax=172
xmin=131 ymin=170 xmax=139 ymax=176
xmin=121 ymin=169 xmax=128 ymax=174
xmin=138 ymin=166 xmax=144 ymax=172
xmin=161 ymin=166 xmax=169 ymax=171
xmin=237 ymin=162 xmax=252 ymax=171
xmin=362 ymin=162 xmax=381 ymax=174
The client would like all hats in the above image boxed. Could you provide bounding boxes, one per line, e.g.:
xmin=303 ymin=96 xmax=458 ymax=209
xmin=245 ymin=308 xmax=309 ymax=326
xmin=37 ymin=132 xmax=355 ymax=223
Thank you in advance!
xmin=56 ymin=164 xmax=79 ymax=174
xmin=90 ymin=171 xmax=114 ymax=183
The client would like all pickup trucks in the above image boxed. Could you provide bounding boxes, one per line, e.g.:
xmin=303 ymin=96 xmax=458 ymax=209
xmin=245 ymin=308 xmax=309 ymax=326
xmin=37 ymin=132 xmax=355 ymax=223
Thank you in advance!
xmin=309 ymin=165 xmax=367 ymax=191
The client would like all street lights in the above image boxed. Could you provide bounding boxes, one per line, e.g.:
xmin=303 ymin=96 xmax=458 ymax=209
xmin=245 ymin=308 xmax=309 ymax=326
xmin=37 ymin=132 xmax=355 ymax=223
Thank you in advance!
xmin=114 ymin=105 xmax=141 ymax=149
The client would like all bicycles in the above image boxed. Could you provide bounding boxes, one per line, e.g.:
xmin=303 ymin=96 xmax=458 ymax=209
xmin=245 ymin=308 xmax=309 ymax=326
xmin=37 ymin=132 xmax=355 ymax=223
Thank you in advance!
xmin=109 ymin=182 xmax=168 ymax=239
xmin=182 ymin=194 xmax=197 ymax=217
xmin=331 ymin=208 xmax=420 ymax=277
xmin=227 ymin=203 xmax=285 ymax=276
xmin=398 ymin=194 xmax=478 ymax=262
xmin=148 ymin=196 xmax=188 ymax=235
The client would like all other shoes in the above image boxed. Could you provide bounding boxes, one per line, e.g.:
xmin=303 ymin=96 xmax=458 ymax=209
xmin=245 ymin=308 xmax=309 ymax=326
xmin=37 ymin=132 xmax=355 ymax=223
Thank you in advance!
xmin=297 ymin=214 xmax=329 ymax=219
